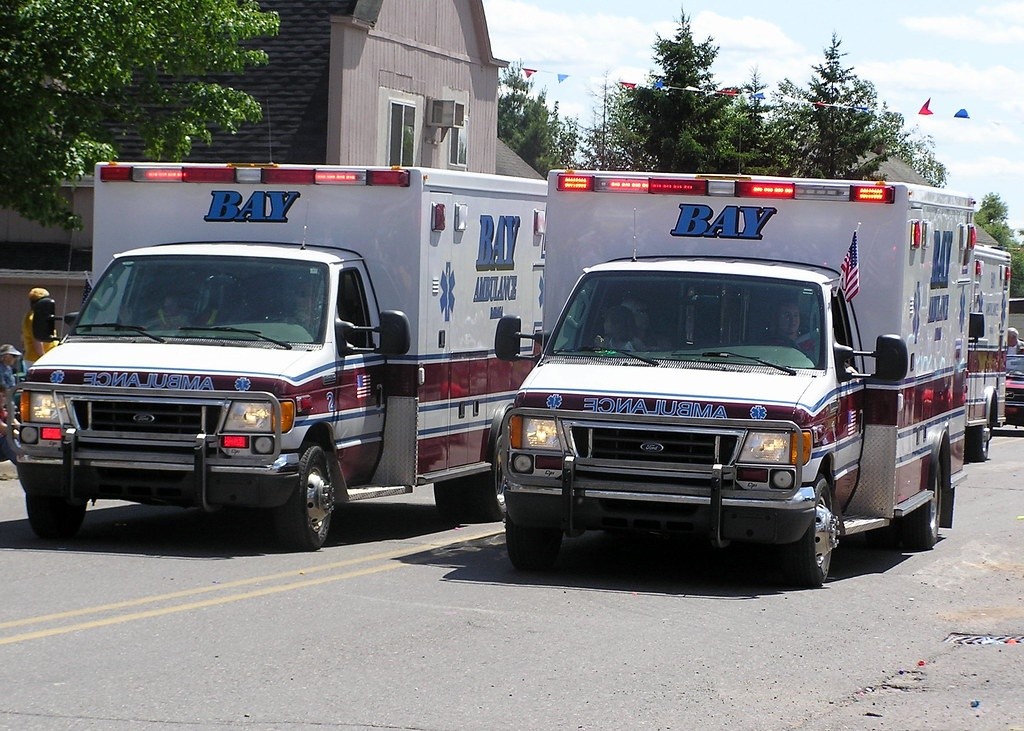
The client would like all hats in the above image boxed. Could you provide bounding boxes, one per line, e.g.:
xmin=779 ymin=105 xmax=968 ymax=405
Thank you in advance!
xmin=0 ymin=344 xmax=22 ymax=356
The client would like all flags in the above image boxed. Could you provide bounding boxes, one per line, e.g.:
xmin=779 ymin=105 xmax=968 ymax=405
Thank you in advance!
xmin=81 ymin=278 xmax=93 ymax=305
xmin=841 ymin=231 xmax=859 ymax=302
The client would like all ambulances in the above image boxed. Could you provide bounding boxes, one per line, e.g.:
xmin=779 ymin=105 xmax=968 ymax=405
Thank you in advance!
xmin=7 ymin=159 xmax=546 ymax=552
xmin=501 ymin=167 xmax=977 ymax=590
xmin=969 ymin=244 xmax=1011 ymax=464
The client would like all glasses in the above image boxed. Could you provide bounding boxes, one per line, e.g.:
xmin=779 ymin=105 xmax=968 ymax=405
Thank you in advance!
xmin=9 ymin=354 xmax=16 ymax=358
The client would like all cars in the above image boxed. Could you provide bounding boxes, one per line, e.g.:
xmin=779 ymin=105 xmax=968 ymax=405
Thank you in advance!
xmin=1002 ymin=355 xmax=1024 ymax=427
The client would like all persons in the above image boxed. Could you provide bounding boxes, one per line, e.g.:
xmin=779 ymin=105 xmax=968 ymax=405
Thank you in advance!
xmin=593 ymin=306 xmax=661 ymax=353
xmin=118 ymin=293 xmax=190 ymax=330
xmin=761 ymin=300 xmax=815 ymax=363
xmin=273 ymin=280 xmax=321 ymax=328
xmin=1007 ymin=327 xmax=1024 ymax=355
xmin=0 ymin=288 xmax=60 ymax=466
